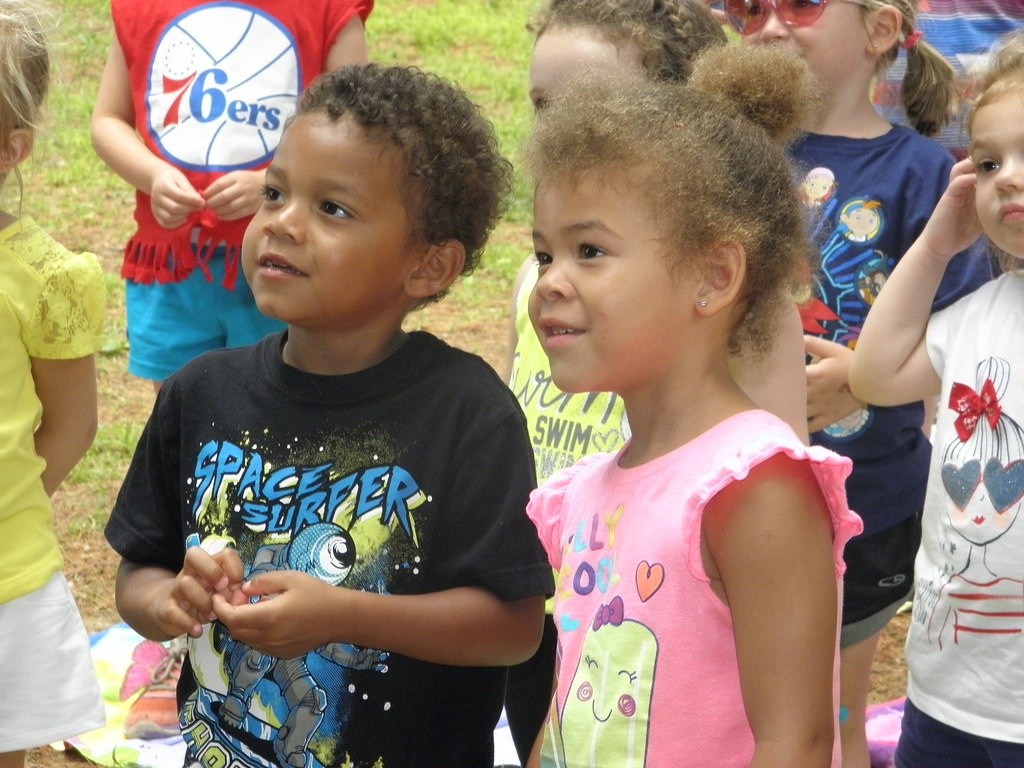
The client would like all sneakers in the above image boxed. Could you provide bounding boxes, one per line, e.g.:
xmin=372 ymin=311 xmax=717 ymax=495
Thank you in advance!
xmin=123 ymin=633 xmax=188 ymax=738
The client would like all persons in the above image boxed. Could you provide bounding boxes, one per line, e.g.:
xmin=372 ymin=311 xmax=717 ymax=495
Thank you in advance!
xmin=848 ymin=25 xmax=1023 ymax=768
xmin=525 ymin=47 xmax=862 ymax=768
xmin=721 ymin=0 xmax=999 ymax=768
xmin=101 ymin=61 xmax=559 ymax=768
xmin=90 ymin=0 xmax=376 ymax=737
xmin=0 ymin=0 xmax=106 ymax=768
xmin=496 ymin=0 xmax=811 ymax=768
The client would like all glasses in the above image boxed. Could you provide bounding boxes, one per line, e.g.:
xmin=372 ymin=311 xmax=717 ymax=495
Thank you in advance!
xmin=723 ymin=0 xmax=827 ymax=35
